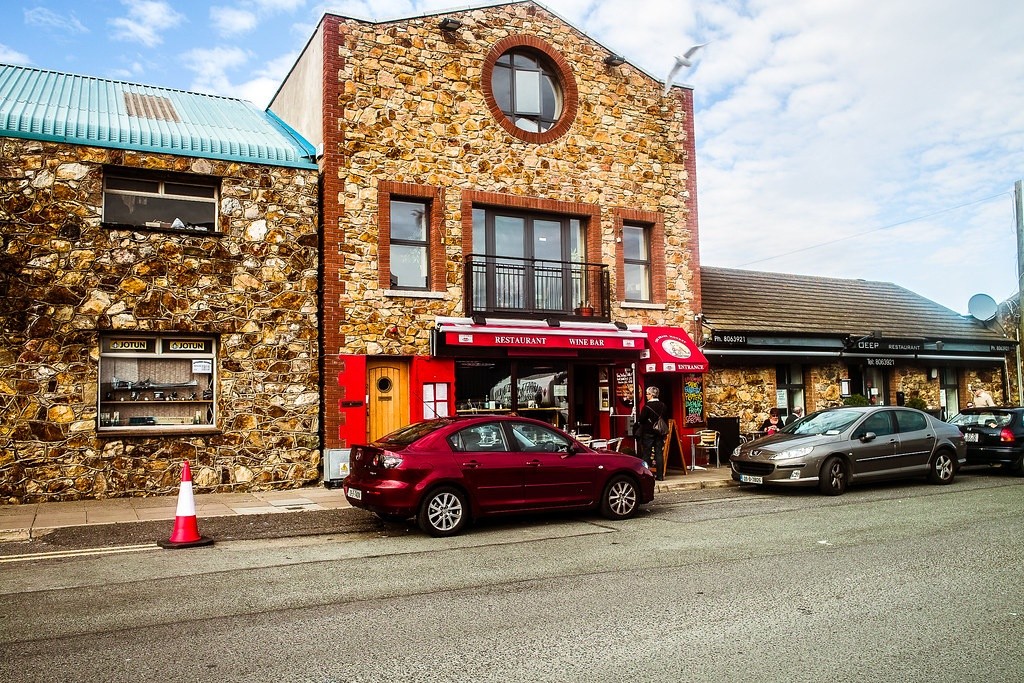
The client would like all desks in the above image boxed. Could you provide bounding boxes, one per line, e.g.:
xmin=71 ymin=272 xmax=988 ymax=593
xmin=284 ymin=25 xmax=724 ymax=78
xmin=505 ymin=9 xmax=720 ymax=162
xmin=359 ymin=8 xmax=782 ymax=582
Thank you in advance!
xmin=683 ymin=434 xmax=708 ymax=471
xmin=455 ymin=407 xmax=566 ymax=428
xmin=740 ymin=431 xmax=765 ymax=441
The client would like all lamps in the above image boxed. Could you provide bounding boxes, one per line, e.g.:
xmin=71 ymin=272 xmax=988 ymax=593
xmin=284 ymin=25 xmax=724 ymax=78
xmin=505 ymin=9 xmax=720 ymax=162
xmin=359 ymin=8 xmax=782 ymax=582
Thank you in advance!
xmin=924 ymin=341 xmax=944 ymax=351
xmin=469 ymin=315 xmax=487 ymax=325
xmin=609 ymin=322 xmax=628 ymax=330
xmin=856 ymin=329 xmax=882 ymax=344
xmin=542 ymin=318 xmax=560 ymax=327
xmin=438 ymin=18 xmax=463 ymax=32
xmin=605 ymin=56 xmax=626 ymax=66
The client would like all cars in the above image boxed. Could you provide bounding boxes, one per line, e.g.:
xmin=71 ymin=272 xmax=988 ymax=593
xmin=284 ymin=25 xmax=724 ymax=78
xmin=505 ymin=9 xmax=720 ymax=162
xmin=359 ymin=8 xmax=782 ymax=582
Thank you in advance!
xmin=947 ymin=406 xmax=1024 ymax=480
xmin=339 ymin=411 xmax=655 ymax=540
xmin=728 ymin=403 xmax=968 ymax=495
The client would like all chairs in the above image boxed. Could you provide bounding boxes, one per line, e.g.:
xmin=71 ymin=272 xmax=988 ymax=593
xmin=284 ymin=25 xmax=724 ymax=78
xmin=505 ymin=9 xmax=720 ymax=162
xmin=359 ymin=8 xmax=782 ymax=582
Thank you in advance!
xmin=692 ymin=430 xmax=722 ymax=468
xmin=575 ymin=434 xmax=592 ymax=448
xmin=867 ymin=418 xmax=888 ymax=435
xmin=985 ymin=419 xmax=996 ymax=426
xmin=462 ymin=432 xmax=483 ymax=451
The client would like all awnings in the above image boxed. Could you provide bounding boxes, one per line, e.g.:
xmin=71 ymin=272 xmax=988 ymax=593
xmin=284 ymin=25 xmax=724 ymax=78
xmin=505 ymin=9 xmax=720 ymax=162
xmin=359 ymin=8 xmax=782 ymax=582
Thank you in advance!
xmin=636 ymin=327 xmax=710 ymax=374
xmin=434 ymin=317 xmax=648 ymax=350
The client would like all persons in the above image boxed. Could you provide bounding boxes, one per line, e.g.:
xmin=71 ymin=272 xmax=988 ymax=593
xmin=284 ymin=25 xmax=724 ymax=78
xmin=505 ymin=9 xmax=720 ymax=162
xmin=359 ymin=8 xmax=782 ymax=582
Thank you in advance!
xmin=971 ymin=381 xmax=995 ymax=408
xmin=762 ymin=408 xmax=784 ymax=436
xmin=638 ymin=386 xmax=669 ymax=481
xmin=783 ymin=406 xmax=803 ymax=426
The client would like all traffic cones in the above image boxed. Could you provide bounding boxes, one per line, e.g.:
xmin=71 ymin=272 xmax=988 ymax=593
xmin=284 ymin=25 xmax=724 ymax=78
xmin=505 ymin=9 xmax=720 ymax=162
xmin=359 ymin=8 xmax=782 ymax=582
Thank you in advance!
xmin=156 ymin=461 xmax=215 ymax=550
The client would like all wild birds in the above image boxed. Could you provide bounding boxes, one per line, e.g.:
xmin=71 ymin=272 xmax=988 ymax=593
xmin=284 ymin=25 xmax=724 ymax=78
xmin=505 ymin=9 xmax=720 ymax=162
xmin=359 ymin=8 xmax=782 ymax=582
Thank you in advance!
xmin=662 ymin=41 xmax=712 ymax=97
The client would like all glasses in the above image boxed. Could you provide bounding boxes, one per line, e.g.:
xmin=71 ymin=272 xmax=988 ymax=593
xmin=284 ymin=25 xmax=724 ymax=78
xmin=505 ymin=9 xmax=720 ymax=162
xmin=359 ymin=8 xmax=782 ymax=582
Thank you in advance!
xmin=772 ymin=414 xmax=778 ymax=417
xmin=972 ymin=390 xmax=977 ymax=392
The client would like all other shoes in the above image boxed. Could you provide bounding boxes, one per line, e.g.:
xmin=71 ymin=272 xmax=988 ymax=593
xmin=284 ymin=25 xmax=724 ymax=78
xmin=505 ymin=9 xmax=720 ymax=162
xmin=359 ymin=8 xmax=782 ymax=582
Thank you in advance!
xmin=655 ymin=477 xmax=663 ymax=481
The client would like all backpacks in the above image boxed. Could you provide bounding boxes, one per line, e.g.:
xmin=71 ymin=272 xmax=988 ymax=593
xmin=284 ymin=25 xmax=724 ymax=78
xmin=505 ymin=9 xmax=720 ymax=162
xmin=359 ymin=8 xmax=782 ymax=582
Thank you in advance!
xmin=644 ymin=402 xmax=670 ymax=436
